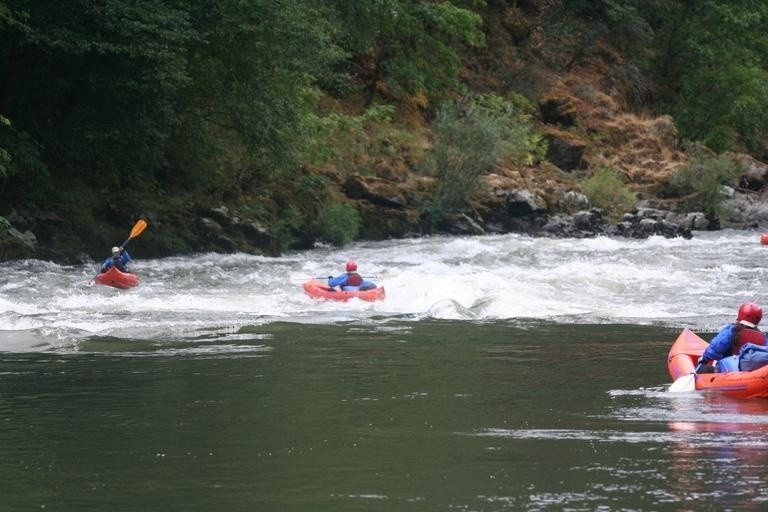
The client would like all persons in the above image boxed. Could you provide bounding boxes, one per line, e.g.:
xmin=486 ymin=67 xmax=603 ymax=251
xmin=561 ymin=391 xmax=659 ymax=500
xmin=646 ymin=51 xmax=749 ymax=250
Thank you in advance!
xmin=328 ymin=261 xmax=363 ymax=291
xmin=101 ymin=246 xmax=131 ymax=273
xmin=697 ymin=302 xmax=768 ymax=374
xmin=760 ymin=233 xmax=768 ymax=247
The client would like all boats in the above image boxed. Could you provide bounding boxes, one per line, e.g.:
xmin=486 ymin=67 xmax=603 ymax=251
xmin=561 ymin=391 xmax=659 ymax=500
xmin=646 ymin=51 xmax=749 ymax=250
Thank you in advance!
xmin=302 ymin=279 xmax=385 ymax=302
xmin=95 ymin=266 xmax=139 ymax=290
xmin=667 ymin=328 xmax=767 ymax=401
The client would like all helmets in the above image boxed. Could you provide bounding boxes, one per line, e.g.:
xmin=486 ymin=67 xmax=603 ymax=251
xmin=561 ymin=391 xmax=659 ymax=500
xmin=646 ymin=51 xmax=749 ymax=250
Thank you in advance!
xmin=112 ymin=247 xmax=119 ymax=253
xmin=738 ymin=304 xmax=763 ymax=325
xmin=346 ymin=262 xmax=357 ymax=271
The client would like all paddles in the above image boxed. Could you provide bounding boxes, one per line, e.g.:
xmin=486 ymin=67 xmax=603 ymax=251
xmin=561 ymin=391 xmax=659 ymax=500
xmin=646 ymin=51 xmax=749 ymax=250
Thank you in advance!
xmin=290 ymin=272 xmax=399 ymax=286
xmin=87 ymin=219 xmax=147 ymax=286
xmin=667 ymin=362 xmax=703 ymax=393
